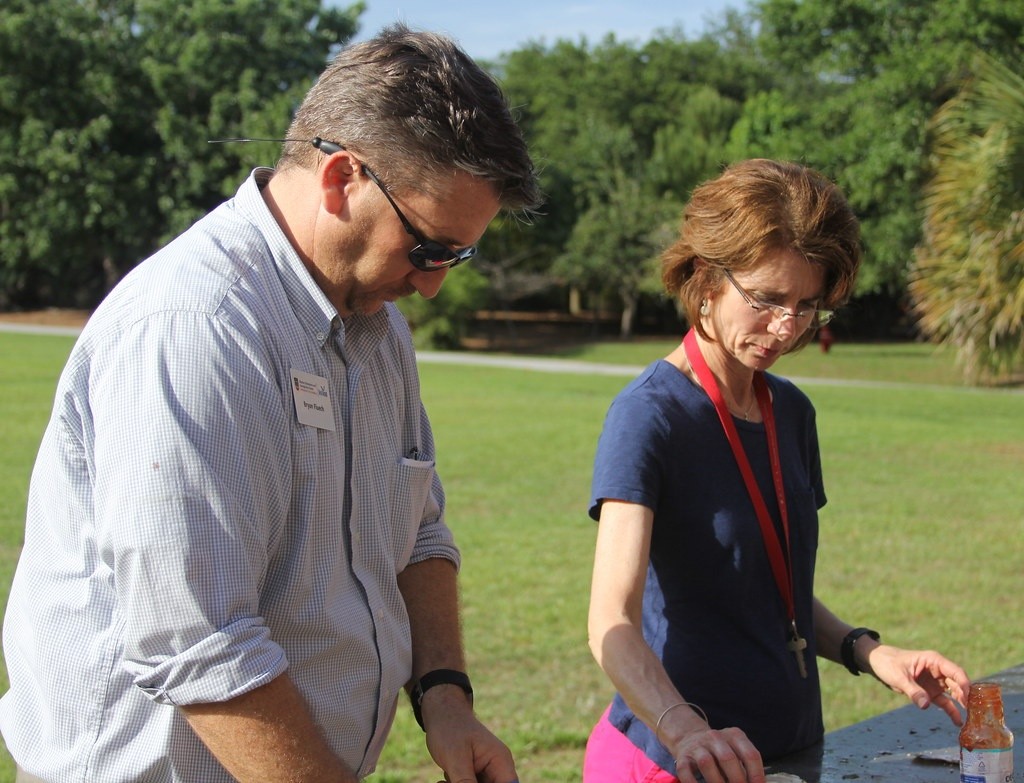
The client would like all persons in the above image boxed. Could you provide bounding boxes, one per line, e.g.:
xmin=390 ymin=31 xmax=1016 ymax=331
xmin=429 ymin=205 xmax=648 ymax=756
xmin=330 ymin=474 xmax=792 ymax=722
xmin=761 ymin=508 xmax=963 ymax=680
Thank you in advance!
xmin=582 ymin=156 xmax=971 ymax=783
xmin=1 ymin=23 xmax=526 ymax=783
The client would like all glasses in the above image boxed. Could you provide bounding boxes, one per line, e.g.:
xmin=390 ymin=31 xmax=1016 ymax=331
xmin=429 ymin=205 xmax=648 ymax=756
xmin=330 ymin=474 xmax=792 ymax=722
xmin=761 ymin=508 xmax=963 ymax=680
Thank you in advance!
xmin=724 ymin=265 xmax=837 ymax=331
xmin=311 ymin=136 xmax=478 ymax=272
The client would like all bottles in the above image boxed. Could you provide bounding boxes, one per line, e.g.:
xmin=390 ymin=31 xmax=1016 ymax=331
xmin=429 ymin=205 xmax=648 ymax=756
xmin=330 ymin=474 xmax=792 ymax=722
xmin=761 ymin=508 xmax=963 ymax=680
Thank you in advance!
xmin=959 ymin=683 xmax=1013 ymax=783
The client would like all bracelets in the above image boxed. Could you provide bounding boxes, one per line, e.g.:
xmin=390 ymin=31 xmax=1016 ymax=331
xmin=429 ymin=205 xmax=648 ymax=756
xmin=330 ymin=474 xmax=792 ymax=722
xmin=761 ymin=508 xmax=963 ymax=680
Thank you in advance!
xmin=654 ymin=699 xmax=709 ymax=754
xmin=407 ymin=665 xmax=475 ymax=732
xmin=840 ymin=626 xmax=880 ymax=677
